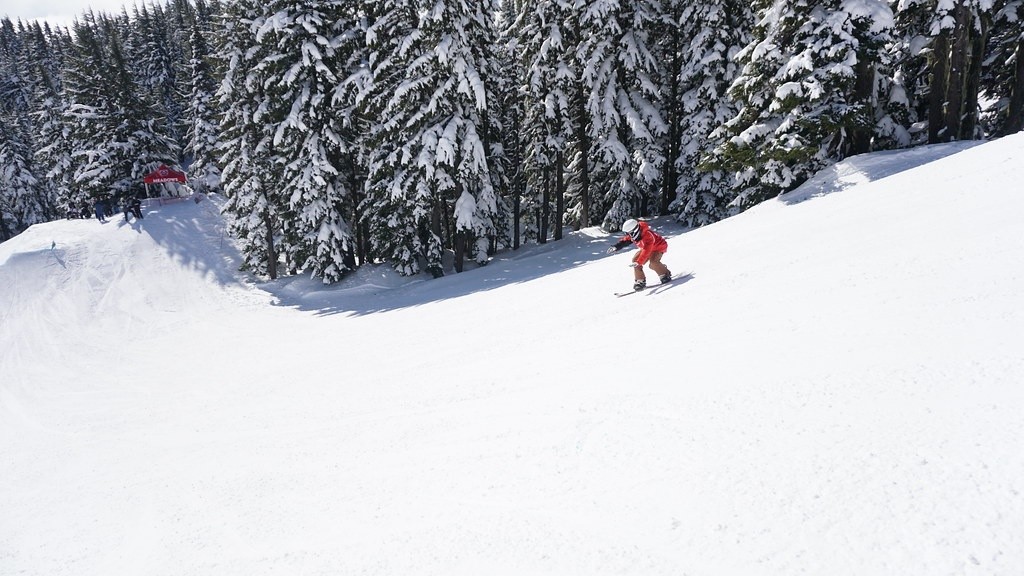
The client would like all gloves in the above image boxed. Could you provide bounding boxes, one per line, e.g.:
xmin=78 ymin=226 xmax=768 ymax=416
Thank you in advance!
xmin=629 ymin=261 xmax=639 ymax=268
xmin=606 ymin=246 xmax=617 ymax=255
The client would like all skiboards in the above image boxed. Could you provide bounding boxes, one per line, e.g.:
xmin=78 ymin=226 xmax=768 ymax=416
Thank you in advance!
xmin=614 ymin=271 xmax=692 ymax=297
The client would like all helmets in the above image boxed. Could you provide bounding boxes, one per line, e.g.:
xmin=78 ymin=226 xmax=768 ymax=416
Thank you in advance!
xmin=622 ymin=219 xmax=640 ymax=241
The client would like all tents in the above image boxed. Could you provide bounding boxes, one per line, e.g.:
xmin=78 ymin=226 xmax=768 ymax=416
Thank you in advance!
xmin=144 ymin=164 xmax=186 ymax=198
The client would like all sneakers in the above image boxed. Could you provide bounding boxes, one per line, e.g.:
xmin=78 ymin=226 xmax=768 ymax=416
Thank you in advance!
xmin=633 ymin=278 xmax=646 ymax=290
xmin=659 ymin=270 xmax=672 ymax=284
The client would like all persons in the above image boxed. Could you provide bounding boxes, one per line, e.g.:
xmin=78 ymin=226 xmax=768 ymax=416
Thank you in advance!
xmin=93 ymin=195 xmax=143 ymax=222
xmin=607 ymin=219 xmax=672 ymax=290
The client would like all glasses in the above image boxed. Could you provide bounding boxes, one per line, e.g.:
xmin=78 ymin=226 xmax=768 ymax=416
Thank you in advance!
xmin=624 ymin=232 xmax=635 ymax=238
